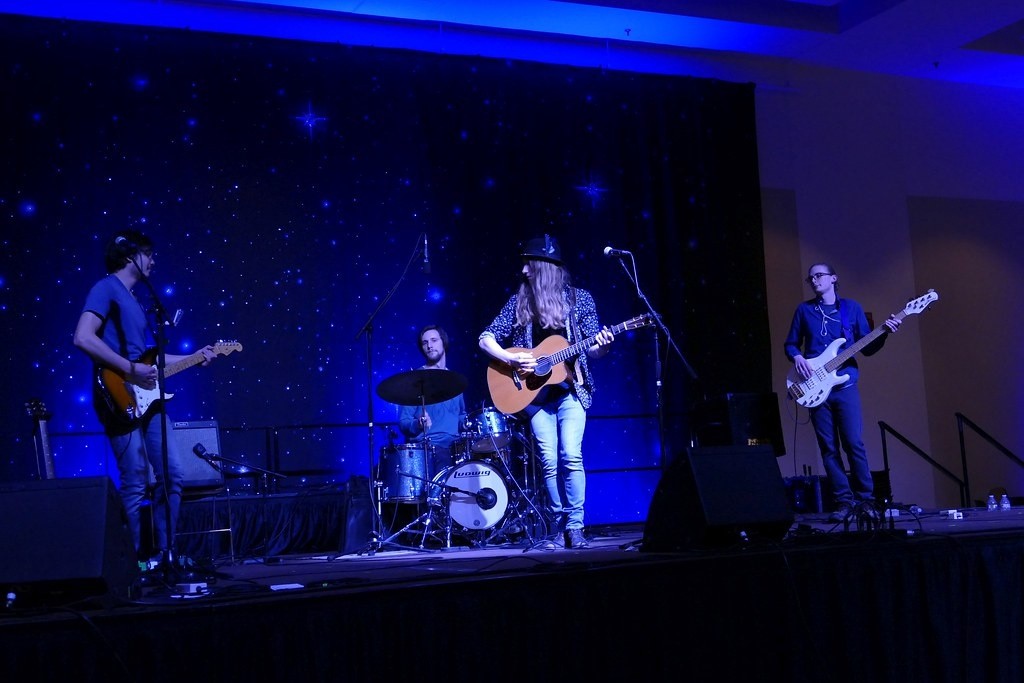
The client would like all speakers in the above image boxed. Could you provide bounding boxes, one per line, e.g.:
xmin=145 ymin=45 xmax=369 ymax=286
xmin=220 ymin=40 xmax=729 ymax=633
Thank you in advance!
xmin=697 ymin=393 xmax=786 ymax=458
xmin=0 ymin=475 xmax=144 ymax=613
xmin=146 ymin=420 xmax=226 ymax=490
xmin=642 ymin=442 xmax=795 ymax=552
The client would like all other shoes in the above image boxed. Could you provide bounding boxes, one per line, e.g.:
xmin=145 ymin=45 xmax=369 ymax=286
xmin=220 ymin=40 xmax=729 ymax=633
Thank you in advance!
xmin=567 ymin=528 xmax=589 ymax=549
xmin=546 ymin=531 xmax=566 ymax=549
xmin=830 ymin=507 xmax=859 ymax=522
xmin=862 ymin=508 xmax=881 ymax=522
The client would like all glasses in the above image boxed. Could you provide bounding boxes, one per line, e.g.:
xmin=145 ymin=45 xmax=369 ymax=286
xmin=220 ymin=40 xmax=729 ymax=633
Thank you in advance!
xmin=805 ymin=273 xmax=834 ymax=284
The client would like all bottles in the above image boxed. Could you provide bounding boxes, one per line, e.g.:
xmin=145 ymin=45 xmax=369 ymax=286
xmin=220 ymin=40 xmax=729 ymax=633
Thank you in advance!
xmin=987 ymin=496 xmax=998 ymax=512
xmin=911 ymin=506 xmax=922 ymax=513
xmin=1000 ymin=495 xmax=1010 ymax=512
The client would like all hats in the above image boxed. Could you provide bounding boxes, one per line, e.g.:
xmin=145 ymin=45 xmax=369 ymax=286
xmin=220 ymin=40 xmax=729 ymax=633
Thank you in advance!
xmin=519 ymin=234 xmax=561 ymax=264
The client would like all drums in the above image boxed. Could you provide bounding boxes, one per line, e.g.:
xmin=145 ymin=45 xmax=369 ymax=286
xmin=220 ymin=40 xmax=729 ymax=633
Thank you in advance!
xmin=461 ymin=405 xmax=513 ymax=452
xmin=450 ymin=435 xmax=512 ymax=468
xmin=428 ymin=458 xmax=512 ymax=533
xmin=378 ymin=442 xmax=437 ymax=504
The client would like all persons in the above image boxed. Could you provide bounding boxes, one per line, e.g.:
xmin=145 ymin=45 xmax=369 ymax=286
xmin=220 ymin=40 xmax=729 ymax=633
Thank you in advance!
xmin=479 ymin=234 xmax=615 ymax=550
xmin=74 ymin=228 xmax=217 ymax=585
xmin=784 ymin=263 xmax=902 ymax=520
xmin=399 ymin=326 xmax=469 ymax=472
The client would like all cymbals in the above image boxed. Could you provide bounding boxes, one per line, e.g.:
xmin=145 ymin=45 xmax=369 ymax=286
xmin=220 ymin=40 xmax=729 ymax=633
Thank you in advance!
xmin=376 ymin=368 xmax=468 ymax=406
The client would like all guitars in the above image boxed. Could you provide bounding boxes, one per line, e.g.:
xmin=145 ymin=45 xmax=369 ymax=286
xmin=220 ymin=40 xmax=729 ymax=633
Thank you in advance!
xmin=785 ymin=287 xmax=942 ymax=409
xmin=486 ymin=311 xmax=655 ymax=419
xmin=96 ymin=338 xmax=244 ymax=423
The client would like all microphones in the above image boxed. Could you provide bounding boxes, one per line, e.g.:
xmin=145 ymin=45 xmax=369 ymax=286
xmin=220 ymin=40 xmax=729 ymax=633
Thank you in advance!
xmin=115 ymin=237 xmax=138 ymax=249
xmin=193 ymin=442 xmax=219 ymax=468
xmin=483 ymin=492 xmax=495 ymax=505
xmin=604 ymin=247 xmax=631 ymax=257
xmin=424 ymin=233 xmax=432 ymax=273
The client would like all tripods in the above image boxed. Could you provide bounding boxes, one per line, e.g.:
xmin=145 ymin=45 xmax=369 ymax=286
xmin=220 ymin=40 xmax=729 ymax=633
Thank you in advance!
xmin=479 ymin=422 xmax=555 ymax=548
xmin=327 ymin=248 xmax=441 ymax=563
